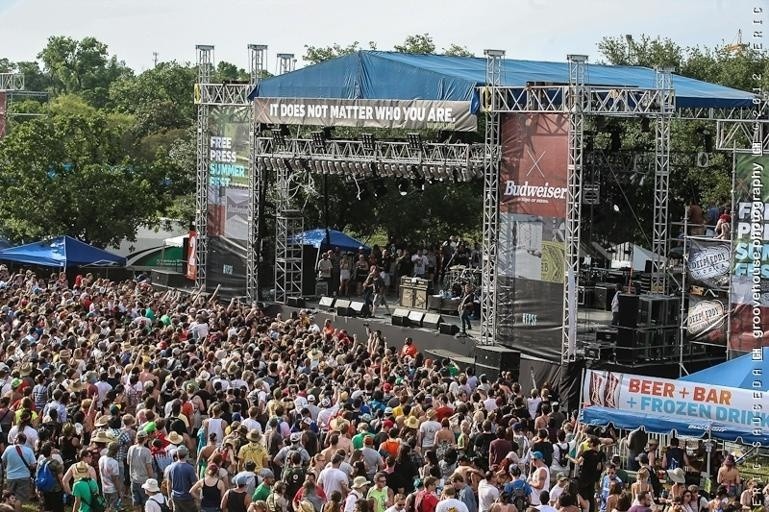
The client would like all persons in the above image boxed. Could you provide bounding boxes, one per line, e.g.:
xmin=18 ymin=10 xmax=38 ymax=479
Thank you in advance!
xmin=0 ymin=198 xmax=769 ymax=511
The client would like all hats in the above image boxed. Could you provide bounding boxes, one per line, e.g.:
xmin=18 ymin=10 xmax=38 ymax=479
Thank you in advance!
xmin=307 ymin=349 xmax=324 ymax=361
xmin=158 ymin=348 xmax=239 ymax=390
xmin=509 ymin=465 xmax=519 ymax=472
xmin=724 ymin=455 xmax=736 ymax=466
xmin=94 ymin=414 xmax=113 ymax=427
xmin=140 ymin=478 xmax=161 ymax=492
xmin=557 ymin=472 xmax=568 ymax=481
xmin=351 ymin=476 xmax=370 ymax=489
xmin=232 ymin=364 xmax=301 ymax=484
xmin=136 ymin=431 xmax=189 ymax=455
xmin=648 ymin=439 xmax=659 ymax=445
xmin=10 ymin=363 xmax=33 ymax=388
xmin=308 ymin=384 xmax=438 ymax=431
xmin=59 ymin=350 xmax=77 ymax=366
xmin=90 ymin=432 xmax=111 ymax=442
xmin=74 ymin=461 xmax=89 ymax=476
xmin=667 ymin=468 xmax=685 ymax=483
xmin=478 ymin=384 xmax=539 ymax=432
xmin=634 ymin=453 xmax=647 ymax=461
xmin=530 ymin=452 xmax=543 ymax=459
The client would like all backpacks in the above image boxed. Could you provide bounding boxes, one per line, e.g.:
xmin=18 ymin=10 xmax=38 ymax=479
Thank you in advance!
xmin=592 ymin=450 xmax=603 ymax=482
xmin=150 ymin=497 xmax=171 ymax=511
xmin=554 ymin=443 xmax=569 ymax=467
xmin=80 ymin=476 xmax=108 ymax=512
xmin=510 ymin=481 xmax=529 ymax=512
xmin=35 ymin=458 xmax=55 ymax=492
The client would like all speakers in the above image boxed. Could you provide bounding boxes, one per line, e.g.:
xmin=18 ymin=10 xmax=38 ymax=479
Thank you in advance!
xmin=399 ymin=277 xmax=434 ymax=311
xmin=333 ymin=299 xmax=351 ymax=316
xmin=150 ymin=269 xmax=219 ymax=302
xmin=313 ymin=280 xmax=329 ymax=296
xmin=439 ymin=323 xmax=460 ymax=335
xmin=285 ymin=297 xmax=307 ymax=308
xmin=407 ymin=311 xmax=425 ymax=327
xmin=423 ymin=345 xmax=521 ymax=385
xmin=575 ymin=268 xmax=708 ymax=365
xmin=391 ymin=309 xmax=410 ymax=326
xmin=318 ymin=297 xmax=335 ymax=312
xmin=422 ymin=313 xmax=442 ymax=329
xmin=348 ymin=302 xmax=369 ymax=318
